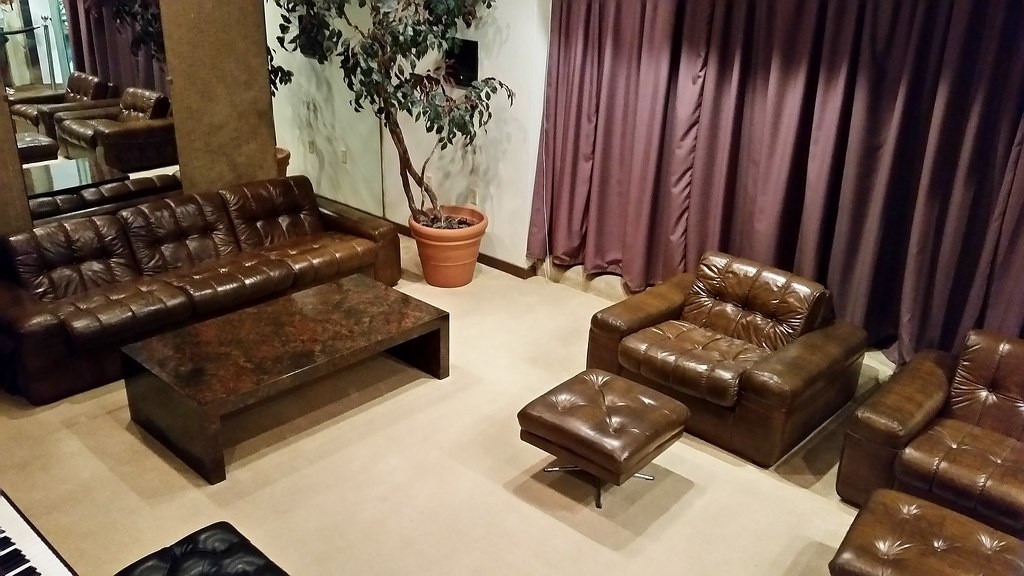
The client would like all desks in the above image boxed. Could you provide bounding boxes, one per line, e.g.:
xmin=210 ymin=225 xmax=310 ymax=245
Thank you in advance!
xmin=21 ymin=157 xmax=129 ymax=201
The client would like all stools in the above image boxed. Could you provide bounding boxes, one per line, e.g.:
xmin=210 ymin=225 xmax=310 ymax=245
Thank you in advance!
xmin=114 ymin=521 xmax=290 ymax=576
xmin=517 ymin=367 xmax=691 ymax=508
xmin=17 ymin=132 xmax=59 ymax=165
xmin=827 ymin=489 xmax=1024 ymax=576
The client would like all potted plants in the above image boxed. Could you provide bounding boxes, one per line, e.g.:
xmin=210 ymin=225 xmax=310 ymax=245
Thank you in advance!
xmin=271 ymin=0 xmax=517 ymax=289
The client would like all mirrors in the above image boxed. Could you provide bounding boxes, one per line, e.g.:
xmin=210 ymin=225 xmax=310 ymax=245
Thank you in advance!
xmin=1 ymin=0 xmax=182 ymax=226
xmin=264 ymin=0 xmax=384 ymax=218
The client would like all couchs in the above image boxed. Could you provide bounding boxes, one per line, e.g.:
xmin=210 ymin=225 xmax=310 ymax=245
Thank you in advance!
xmin=52 ymin=85 xmax=178 ymax=173
xmin=836 ymin=327 xmax=1024 ymax=540
xmin=584 ymin=250 xmax=867 ymax=469
xmin=7 ymin=71 xmax=127 ymax=141
xmin=0 ymin=172 xmax=402 ymax=409
xmin=27 ymin=168 xmax=182 ymax=225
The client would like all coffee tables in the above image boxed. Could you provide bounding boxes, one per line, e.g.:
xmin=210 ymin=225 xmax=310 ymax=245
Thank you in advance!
xmin=119 ymin=273 xmax=450 ymax=487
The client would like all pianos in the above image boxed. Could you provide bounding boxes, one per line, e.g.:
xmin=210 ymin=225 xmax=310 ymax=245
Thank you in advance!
xmin=0 ymin=486 xmax=81 ymax=576
